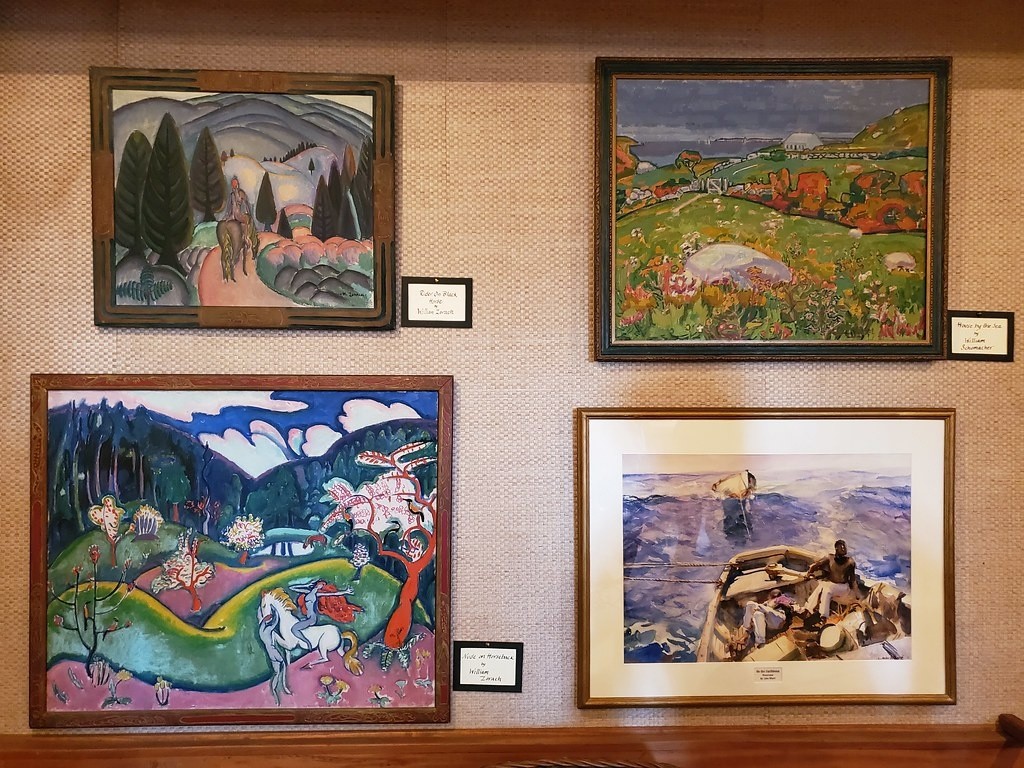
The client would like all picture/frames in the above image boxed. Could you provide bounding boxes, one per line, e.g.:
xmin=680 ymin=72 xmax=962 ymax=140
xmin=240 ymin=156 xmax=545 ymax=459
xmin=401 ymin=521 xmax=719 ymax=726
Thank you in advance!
xmin=452 ymin=639 xmax=524 ymax=693
xmin=591 ymin=52 xmax=950 ymax=362
xmin=87 ymin=63 xmax=397 ymax=330
xmin=401 ymin=274 xmax=474 ymax=328
xmin=575 ymin=406 xmax=959 ymax=707
xmin=948 ymin=305 xmax=1015 ymax=364
xmin=27 ymin=371 xmax=454 ymax=725
